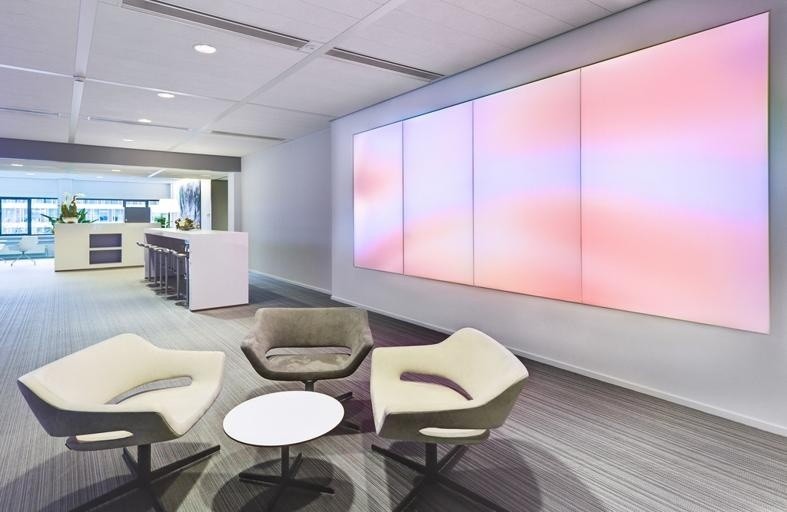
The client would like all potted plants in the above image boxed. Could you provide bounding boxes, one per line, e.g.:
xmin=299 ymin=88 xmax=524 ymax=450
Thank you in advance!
xmin=60 ymin=194 xmax=80 ymax=224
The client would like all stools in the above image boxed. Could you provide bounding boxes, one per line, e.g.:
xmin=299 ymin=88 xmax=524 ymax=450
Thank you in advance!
xmin=135 ymin=240 xmax=188 ymax=308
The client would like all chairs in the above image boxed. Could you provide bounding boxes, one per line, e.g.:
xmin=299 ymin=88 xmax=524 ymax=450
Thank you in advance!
xmin=240 ymin=305 xmax=373 ymax=432
xmin=15 ymin=330 xmax=227 ymax=511
xmin=11 ymin=235 xmax=39 ymax=266
xmin=366 ymin=325 xmax=530 ymax=511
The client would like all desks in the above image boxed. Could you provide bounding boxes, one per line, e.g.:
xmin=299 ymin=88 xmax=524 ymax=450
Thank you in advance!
xmin=221 ymin=391 xmax=346 ymax=512
